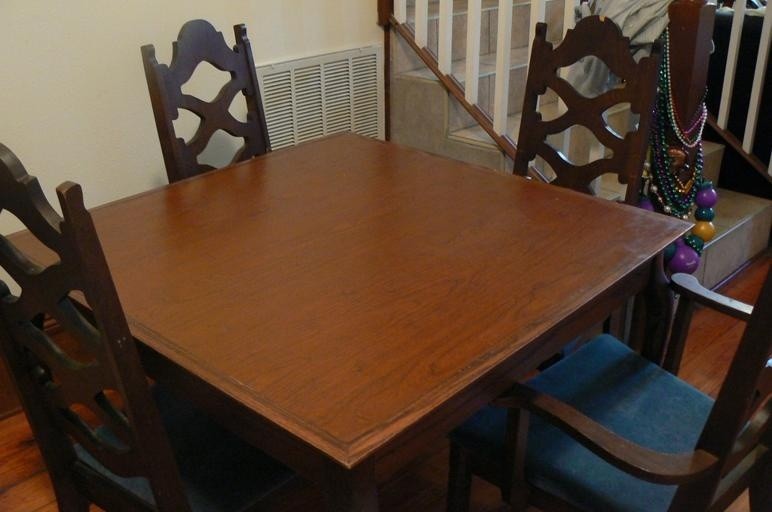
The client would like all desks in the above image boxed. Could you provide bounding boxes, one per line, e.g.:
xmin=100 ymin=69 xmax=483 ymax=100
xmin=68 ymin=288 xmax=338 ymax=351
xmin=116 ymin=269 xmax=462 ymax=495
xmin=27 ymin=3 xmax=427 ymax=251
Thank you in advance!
xmin=0 ymin=130 xmax=698 ymax=512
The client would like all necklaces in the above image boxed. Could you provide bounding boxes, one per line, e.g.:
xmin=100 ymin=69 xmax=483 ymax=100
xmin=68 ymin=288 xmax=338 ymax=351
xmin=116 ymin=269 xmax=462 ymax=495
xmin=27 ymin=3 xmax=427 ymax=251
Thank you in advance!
xmin=638 ymin=25 xmax=718 ymax=273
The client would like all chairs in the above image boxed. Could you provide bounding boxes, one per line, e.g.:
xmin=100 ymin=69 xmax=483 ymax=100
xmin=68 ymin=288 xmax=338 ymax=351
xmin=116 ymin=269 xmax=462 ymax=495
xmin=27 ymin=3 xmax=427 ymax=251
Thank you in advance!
xmin=442 ymin=261 xmax=772 ymax=512
xmin=448 ymin=15 xmax=662 ymax=512
xmin=138 ymin=19 xmax=272 ymax=185
xmin=0 ymin=142 xmax=308 ymax=512
